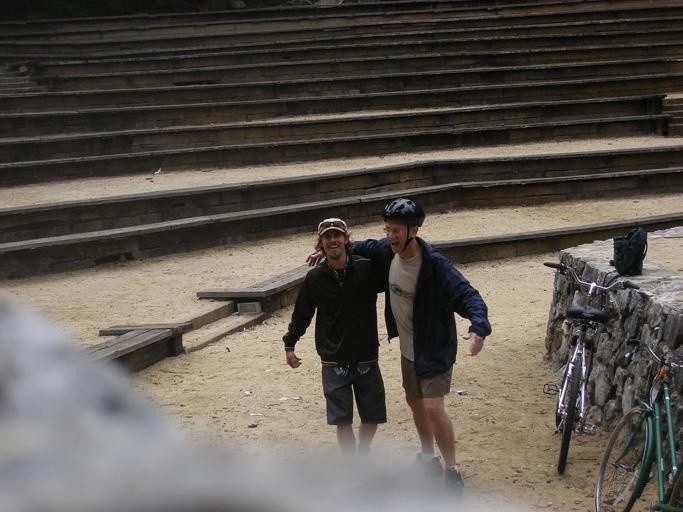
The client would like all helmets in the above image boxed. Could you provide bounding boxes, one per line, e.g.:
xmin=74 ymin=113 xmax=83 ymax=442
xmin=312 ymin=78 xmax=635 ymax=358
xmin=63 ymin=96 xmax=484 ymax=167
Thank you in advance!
xmin=383 ymin=198 xmax=425 ymax=227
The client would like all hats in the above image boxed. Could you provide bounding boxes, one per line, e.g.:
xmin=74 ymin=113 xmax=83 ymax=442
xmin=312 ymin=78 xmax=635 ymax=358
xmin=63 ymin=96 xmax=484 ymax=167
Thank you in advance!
xmin=318 ymin=218 xmax=347 ymax=236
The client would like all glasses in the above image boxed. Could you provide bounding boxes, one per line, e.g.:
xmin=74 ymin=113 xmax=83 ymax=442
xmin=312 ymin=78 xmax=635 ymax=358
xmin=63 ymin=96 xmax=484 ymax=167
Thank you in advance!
xmin=319 ymin=221 xmax=346 ymax=229
xmin=383 ymin=228 xmax=402 ymax=234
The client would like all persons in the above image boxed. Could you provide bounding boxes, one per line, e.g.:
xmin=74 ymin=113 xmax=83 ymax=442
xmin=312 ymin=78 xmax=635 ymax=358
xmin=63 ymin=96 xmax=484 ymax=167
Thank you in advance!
xmin=282 ymin=216 xmax=389 ymax=460
xmin=305 ymin=198 xmax=493 ymax=492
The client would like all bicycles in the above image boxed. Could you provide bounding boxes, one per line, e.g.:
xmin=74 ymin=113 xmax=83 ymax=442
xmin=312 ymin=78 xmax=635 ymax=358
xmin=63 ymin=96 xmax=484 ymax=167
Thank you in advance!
xmin=593 ymin=337 xmax=683 ymax=512
xmin=543 ymin=261 xmax=640 ymax=473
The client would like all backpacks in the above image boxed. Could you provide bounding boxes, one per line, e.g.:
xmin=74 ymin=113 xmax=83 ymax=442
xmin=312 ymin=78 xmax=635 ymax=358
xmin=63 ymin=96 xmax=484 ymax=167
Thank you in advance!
xmin=613 ymin=228 xmax=648 ymax=277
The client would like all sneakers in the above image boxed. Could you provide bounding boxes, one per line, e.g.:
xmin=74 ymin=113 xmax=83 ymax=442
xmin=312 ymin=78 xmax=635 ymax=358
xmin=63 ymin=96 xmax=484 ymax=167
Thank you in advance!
xmin=416 ymin=452 xmax=464 ymax=492
